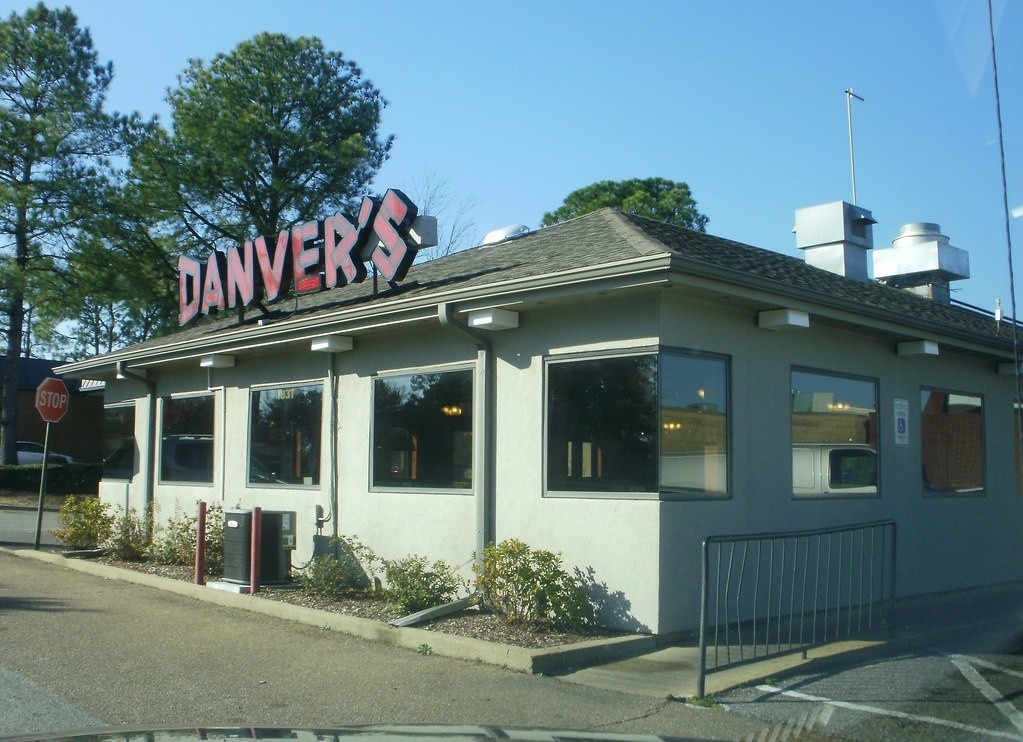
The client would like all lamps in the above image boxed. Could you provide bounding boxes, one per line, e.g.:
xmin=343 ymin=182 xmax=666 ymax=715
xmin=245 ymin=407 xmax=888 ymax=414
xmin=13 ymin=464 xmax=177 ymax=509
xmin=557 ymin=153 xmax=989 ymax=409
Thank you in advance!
xmin=310 ymin=336 xmax=354 ymax=354
xmin=441 ymin=404 xmax=464 ymax=415
xmin=200 ymin=355 xmax=235 ymax=369
xmin=836 ymin=401 xmax=843 ymax=409
xmin=758 ymin=309 xmax=809 ymax=332
xmin=468 ymin=308 xmax=519 ymax=331
xmin=897 ymin=341 xmax=940 ymax=358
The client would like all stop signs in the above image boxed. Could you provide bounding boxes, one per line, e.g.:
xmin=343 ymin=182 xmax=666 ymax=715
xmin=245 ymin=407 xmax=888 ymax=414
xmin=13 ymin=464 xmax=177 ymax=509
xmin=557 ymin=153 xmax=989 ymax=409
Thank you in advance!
xmin=36 ymin=376 xmax=69 ymax=423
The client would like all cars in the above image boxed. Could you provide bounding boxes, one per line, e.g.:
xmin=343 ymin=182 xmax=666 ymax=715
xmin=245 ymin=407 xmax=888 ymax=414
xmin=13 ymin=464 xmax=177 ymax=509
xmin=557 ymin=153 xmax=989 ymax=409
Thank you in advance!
xmin=16 ymin=441 xmax=88 ymax=466
xmin=105 ymin=431 xmax=312 ymax=489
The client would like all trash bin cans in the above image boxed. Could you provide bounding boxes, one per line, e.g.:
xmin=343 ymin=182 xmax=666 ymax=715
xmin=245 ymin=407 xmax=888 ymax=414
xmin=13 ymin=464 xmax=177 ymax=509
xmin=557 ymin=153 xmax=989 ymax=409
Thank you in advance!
xmin=222 ymin=510 xmax=297 ymax=585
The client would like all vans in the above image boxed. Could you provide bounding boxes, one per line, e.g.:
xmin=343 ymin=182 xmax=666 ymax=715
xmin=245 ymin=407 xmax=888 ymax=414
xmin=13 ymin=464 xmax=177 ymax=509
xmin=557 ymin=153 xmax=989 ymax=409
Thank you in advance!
xmin=789 ymin=441 xmax=878 ymax=494
